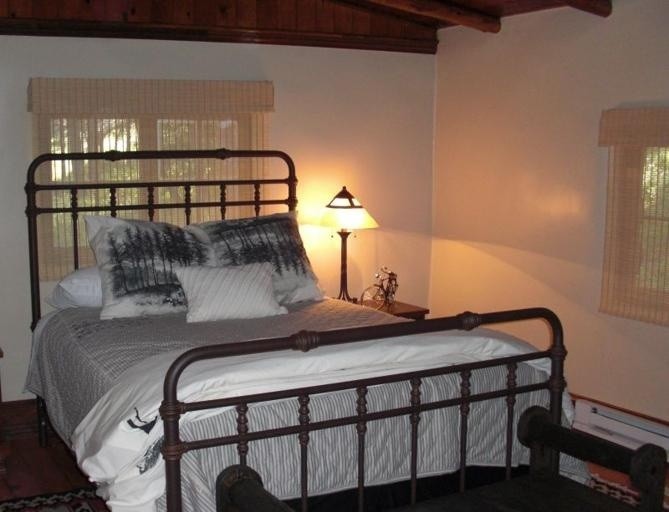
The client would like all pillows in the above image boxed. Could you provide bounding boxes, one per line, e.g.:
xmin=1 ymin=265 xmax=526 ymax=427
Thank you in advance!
xmin=173 ymin=262 xmax=290 ymax=323
xmin=196 ymin=211 xmax=329 ymax=306
xmin=81 ymin=214 xmax=220 ymax=319
xmin=44 ymin=265 xmax=103 ymax=310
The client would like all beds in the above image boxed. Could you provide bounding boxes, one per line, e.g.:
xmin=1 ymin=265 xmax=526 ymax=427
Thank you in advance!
xmin=23 ymin=144 xmax=569 ymax=510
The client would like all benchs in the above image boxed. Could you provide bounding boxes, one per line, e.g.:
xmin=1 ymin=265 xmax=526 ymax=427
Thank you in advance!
xmin=215 ymin=405 xmax=668 ymax=512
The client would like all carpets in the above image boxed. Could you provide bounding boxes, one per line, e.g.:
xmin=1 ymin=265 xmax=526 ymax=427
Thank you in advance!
xmin=7 ymin=474 xmax=669 ymax=512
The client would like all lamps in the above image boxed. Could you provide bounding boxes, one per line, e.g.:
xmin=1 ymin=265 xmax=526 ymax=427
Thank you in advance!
xmin=300 ymin=186 xmax=380 ymax=304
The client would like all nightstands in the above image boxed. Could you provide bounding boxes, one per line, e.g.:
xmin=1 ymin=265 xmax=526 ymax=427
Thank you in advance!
xmin=355 ymin=299 xmax=430 ymax=320
